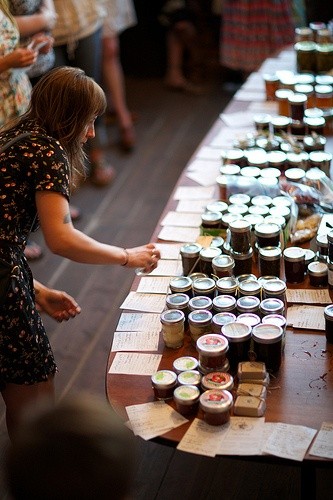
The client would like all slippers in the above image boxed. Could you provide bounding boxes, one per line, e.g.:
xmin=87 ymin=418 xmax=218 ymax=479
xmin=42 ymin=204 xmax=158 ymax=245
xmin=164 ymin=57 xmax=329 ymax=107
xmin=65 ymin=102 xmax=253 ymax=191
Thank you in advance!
xmin=161 ymin=75 xmax=204 ymax=96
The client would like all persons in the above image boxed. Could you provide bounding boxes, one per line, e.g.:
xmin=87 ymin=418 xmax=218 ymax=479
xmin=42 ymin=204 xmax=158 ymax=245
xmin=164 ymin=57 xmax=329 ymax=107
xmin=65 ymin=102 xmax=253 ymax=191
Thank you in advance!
xmin=0 ymin=0 xmax=223 ymax=261
xmin=0 ymin=388 xmax=138 ymax=500
xmin=1 ymin=67 xmax=160 ymax=447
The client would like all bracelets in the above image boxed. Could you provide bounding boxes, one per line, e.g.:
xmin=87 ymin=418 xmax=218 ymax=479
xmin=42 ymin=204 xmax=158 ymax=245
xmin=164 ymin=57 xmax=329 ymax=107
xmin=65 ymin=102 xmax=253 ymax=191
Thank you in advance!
xmin=120 ymin=249 xmax=129 ymax=266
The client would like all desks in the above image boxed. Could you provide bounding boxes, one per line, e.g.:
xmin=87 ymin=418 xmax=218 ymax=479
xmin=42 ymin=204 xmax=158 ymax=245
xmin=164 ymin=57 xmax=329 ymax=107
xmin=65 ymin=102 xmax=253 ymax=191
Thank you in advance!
xmin=106 ymin=43 xmax=333 ymax=473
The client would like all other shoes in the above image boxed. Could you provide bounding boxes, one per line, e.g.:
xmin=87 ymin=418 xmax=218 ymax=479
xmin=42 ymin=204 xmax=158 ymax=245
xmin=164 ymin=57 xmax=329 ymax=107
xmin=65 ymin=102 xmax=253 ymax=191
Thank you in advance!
xmin=121 ymin=127 xmax=137 ymax=149
xmin=24 ymin=241 xmax=45 ymax=260
xmin=105 ymin=108 xmax=139 ymax=124
xmin=90 ymin=150 xmax=115 ymax=185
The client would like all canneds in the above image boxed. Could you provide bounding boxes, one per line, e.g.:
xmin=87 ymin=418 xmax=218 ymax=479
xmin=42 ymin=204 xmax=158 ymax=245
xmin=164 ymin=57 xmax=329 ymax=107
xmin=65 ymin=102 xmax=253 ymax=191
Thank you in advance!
xmin=254 ymin=22 xmax=333 ymax=139
xmin=179 ymin=133 xmax=333 ymax=290
xmin=323 ymin=304 xmax=333 ymax=341
xmin=151 ymin=273 xmax=287 ymax=426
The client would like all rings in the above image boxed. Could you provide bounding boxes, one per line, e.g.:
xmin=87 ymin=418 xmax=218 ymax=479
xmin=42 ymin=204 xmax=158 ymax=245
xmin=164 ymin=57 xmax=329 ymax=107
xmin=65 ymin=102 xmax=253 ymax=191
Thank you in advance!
xmin=151 ymin=254 xmax=156 ymax=260
xmin=151 ymin=249 xmax=156 ymax=253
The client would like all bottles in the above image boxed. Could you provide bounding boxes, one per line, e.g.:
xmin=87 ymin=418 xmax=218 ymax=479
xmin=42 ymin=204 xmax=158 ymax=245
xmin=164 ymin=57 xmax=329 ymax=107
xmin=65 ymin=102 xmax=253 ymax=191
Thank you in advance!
xmin=146 ymin=67 xmax=333 ymax=429
